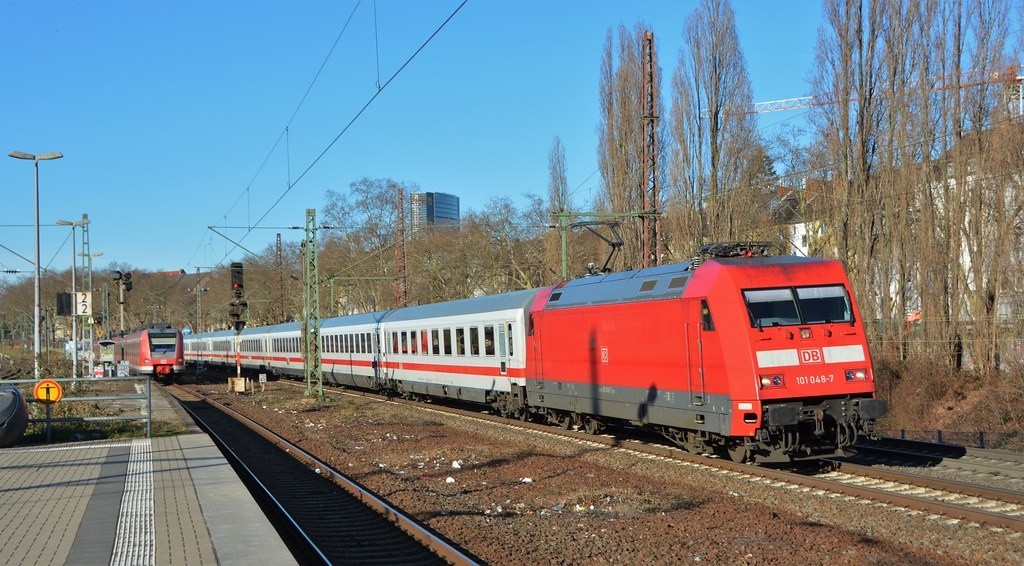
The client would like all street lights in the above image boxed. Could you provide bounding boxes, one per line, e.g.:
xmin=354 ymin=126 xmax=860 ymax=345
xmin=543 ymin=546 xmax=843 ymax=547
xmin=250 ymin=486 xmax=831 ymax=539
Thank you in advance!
xmin=56 ymin=219 xmax=92 ymax=392
xmin=77 ymin=252 xmax=104 ymax=295
xmin=7 ymin=150 xmax=65 ymax=395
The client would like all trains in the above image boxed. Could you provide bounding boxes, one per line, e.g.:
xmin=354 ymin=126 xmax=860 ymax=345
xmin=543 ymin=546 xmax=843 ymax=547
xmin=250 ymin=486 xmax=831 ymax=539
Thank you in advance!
xmin=186 ymin=216 xmax=892 ymax=473
xmin=113 ymin=323 xmax=185 ymax=382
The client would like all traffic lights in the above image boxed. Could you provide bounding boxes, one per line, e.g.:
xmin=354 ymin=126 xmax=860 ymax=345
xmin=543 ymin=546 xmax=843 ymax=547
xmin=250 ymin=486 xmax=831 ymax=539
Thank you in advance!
xmin=233 ymin=320 xmax=246 ymax=331
xmin=231 ymin=262 xmax=246 ymax=294
